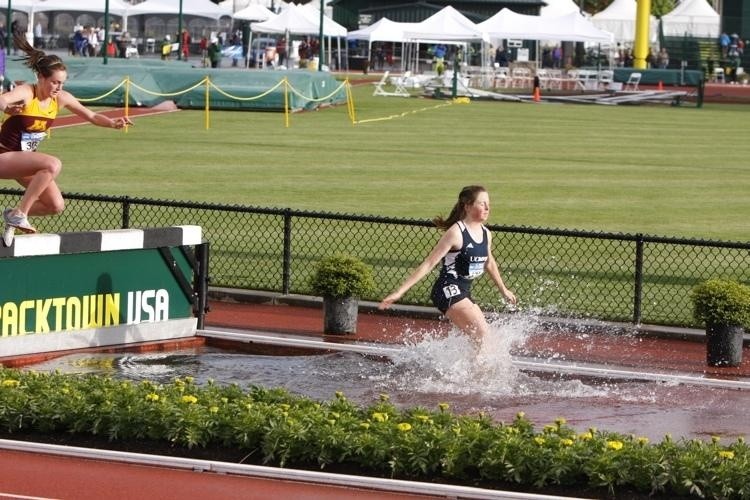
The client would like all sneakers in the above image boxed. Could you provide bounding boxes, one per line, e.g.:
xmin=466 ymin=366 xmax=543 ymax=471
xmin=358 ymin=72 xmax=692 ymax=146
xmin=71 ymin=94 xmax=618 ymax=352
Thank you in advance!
xmin=6 ymin=210 xmax=38 ymax=235
xmin=1 ymin=207 xmax=17 ymax=248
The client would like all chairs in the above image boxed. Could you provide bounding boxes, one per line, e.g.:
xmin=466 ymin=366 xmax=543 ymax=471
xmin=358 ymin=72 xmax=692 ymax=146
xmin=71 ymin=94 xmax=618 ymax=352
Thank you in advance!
xmin=713 ymin=67 xmax=724 ymax=83
xmin=459 ymin=65 xmax=614 ymax=91
xmin=371 ymin=71 xmax=390 ymax=97
xmin=391 ymin=70 xmax=411 ymax=96
xmin=624 ymin=72 xmax=644 ymax=91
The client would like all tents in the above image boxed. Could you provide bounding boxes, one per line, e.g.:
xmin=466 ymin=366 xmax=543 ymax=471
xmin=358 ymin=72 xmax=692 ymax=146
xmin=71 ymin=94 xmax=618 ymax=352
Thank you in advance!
xmin=352 ymin=0 xmax=722 ymax=82
xmin=247 ymin=3 xmax=347 ymax=70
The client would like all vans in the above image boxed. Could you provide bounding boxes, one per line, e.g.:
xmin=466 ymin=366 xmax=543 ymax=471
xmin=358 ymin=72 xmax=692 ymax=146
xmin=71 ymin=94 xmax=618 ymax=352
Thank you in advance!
xmin=251 ymin=37 xmax=277 ymax=58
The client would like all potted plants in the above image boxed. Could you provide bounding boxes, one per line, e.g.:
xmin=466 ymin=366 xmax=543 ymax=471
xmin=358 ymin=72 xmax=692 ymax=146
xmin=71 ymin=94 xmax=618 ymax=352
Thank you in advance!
xmin=306 ymin=254 xmax=376 ymax=336
xmin=689 ymin=280 xmax=750 ymax=366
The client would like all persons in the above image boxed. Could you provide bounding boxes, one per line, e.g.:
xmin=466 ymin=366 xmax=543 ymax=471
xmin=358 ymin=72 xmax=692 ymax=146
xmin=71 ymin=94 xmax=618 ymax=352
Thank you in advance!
xmin=160 ymin=28 xmax=242 ymax=68
xmin=377 ymin=184 xmax=519 ymax=382
xmin=720 ymin=32 xmax=745 ymax=75
xmin=2 ymin=18 xmax=129 ymax=60
xmin=0 ymin=18 xmax=135 ymax=235
xmin=275 ymin=34 xmax=321 ymax=68
